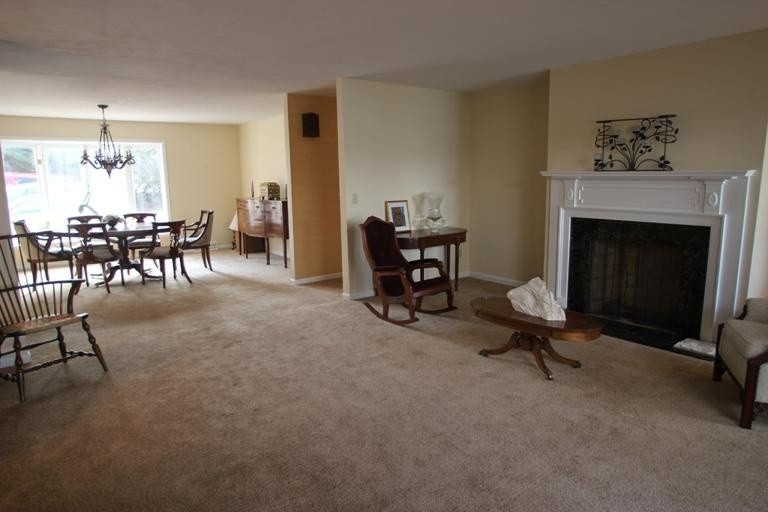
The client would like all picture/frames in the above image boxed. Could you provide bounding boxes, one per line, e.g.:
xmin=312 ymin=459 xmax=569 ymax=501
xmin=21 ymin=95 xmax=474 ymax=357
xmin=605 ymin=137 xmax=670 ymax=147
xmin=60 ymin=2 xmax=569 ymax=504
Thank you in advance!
xmin=384 ymin=199 xmax=412 ymax=232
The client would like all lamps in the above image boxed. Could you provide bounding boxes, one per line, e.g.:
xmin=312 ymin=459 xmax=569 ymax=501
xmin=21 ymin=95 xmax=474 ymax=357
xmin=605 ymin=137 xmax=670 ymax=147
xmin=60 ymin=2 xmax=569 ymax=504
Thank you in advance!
xmin=79 ymin=104 xmax=137 ymax=178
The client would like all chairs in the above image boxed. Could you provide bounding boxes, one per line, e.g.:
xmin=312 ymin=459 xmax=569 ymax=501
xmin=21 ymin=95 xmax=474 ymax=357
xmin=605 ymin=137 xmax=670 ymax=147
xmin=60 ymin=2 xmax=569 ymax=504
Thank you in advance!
xmin=1 ymin=231 xmax=109 ymax=402
xmin=0 ymin=202 xmax=211 ymax=296
xmin=708 ymin=297 xmax=767 ymax=429
xmin=357 ymin=214 xmax=457 ymax=325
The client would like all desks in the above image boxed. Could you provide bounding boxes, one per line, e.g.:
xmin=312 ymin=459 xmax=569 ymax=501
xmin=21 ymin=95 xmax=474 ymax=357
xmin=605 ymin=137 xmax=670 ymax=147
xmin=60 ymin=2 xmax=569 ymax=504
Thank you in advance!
xmin=470 ymin=297 xmax=604 ymax=381
xmin=392 ymin=226 xmax=467 ymax=291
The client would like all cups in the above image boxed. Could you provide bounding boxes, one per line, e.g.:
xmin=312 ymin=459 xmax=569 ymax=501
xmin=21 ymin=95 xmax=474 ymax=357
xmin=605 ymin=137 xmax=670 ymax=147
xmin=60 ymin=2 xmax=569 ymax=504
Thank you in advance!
xmin=424 ymin=191 xmax=446 ymax=233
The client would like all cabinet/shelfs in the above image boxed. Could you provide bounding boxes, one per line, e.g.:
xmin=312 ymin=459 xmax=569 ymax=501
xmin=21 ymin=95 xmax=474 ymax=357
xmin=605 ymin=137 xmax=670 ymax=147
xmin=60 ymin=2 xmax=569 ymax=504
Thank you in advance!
xmin=236 ymin=198 xmax=289 ymax=269
